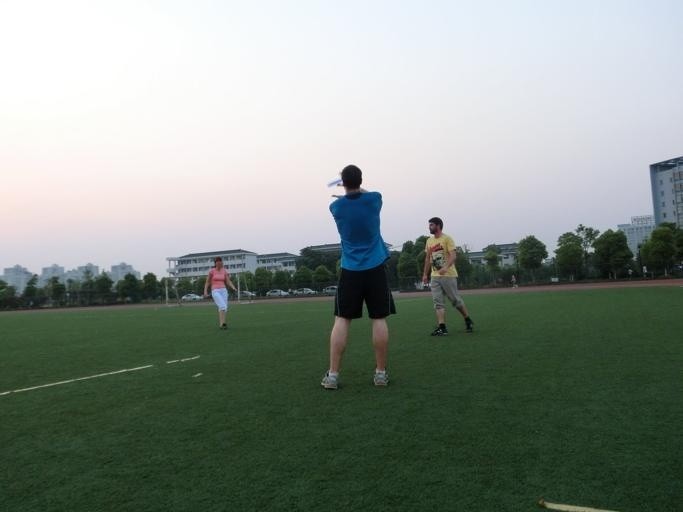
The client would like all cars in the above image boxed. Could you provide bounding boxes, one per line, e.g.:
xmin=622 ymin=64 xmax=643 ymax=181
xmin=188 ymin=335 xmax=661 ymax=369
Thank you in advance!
xmin=323 ymin=286 xmax=339 ymax=296
xmin=237 ymin=290 xmax=257 ymax=298
xmin=266 ymin=289 xmax=291 ymax=297
xmin=182 ymin=293 xmax=204 ymax=302
xmin=291 ymin=288 xmax=318 ymax=297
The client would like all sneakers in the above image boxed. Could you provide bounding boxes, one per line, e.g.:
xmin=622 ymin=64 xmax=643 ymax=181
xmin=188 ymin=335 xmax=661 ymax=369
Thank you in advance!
xmin=432 ymin=325 xmax=448 ymax=336
xmin=321 ymin=370 xmax=338 ymax=390
xmin=466 ymin=320 xmax=473 ymax=334
xmin=373 ymin=370 xmax=390 ymax=386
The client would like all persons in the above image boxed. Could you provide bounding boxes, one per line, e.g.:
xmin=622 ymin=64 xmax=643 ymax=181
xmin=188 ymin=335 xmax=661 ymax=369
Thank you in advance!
xmin=202 ymin=256 xmax=238 ymax=330
xmin=420 ymin=216 xmax=474 ymax=336
xmin=318 ymin=164 xmax=397 ymax=391
